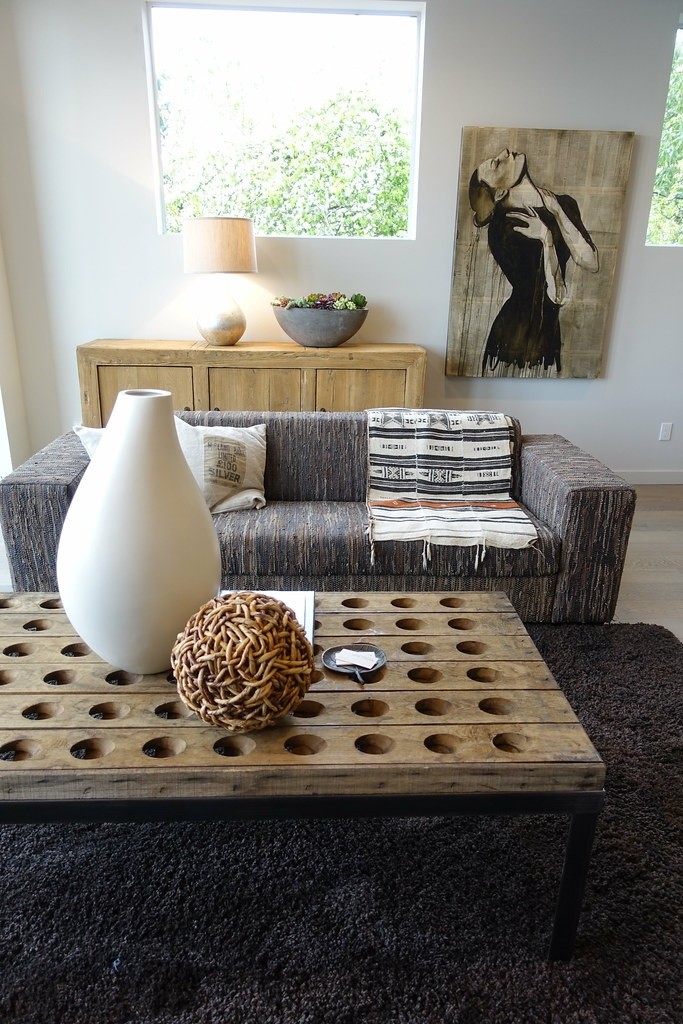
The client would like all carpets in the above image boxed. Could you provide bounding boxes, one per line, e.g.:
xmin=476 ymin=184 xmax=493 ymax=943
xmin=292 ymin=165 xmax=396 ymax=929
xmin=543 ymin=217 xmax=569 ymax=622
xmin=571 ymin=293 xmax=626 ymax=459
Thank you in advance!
xmin=0 ymin=622 xmax=683 ymax=1024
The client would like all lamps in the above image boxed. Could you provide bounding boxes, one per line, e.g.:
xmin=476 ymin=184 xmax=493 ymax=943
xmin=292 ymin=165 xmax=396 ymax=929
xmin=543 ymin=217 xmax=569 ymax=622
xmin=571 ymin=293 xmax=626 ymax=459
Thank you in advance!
xmin=181 ymin=216 xmax=258 ymax=345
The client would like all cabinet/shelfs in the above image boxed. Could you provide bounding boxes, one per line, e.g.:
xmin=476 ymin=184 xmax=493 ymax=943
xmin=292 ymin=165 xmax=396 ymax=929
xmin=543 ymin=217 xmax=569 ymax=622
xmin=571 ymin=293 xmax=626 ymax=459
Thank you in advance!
xmin=78 ymin=338 xmax=428 ymax=428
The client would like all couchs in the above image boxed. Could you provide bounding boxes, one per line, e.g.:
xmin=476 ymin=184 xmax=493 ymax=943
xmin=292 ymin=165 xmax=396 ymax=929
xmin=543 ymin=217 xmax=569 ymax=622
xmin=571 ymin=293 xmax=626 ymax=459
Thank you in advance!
xmin=0 ymin=412 xmax=636 ymax=624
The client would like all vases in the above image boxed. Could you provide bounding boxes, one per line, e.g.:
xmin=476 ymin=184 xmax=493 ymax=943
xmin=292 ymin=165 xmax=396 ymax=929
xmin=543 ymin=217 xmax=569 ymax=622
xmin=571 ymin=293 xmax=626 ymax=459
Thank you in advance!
xmin=56 ymin=387 xmax=223 ymax=679
xmin=272 ymin=305 xmax=368 ymax=347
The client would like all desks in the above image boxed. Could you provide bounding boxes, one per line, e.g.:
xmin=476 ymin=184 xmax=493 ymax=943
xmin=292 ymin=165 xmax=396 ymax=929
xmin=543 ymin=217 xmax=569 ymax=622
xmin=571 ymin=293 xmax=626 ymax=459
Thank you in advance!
xmin=0 ymin=589 xmax=605 ymax=961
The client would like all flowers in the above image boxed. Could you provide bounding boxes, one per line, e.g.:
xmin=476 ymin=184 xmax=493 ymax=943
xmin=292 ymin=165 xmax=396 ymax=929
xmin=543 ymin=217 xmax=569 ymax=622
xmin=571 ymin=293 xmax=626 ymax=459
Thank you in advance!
xmin=271 ymin=292 xmax=369 ymax=311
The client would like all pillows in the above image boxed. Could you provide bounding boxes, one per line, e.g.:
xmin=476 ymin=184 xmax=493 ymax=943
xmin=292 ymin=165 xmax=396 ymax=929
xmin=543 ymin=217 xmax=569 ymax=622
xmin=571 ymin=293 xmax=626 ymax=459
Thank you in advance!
xmin=171 ymin=413 xmax=267 ymax=514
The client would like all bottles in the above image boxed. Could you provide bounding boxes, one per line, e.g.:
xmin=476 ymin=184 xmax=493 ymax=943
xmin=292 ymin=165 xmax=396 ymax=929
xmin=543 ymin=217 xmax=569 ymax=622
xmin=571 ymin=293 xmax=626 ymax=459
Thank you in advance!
xmin=57 ymin=388 xmax=221 ymax=675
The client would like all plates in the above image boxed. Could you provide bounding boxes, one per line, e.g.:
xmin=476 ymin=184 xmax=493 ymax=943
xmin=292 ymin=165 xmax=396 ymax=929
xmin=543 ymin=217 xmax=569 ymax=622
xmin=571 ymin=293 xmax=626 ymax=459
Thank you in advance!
xmin=322 ymin=644 xmax=388 ymax=685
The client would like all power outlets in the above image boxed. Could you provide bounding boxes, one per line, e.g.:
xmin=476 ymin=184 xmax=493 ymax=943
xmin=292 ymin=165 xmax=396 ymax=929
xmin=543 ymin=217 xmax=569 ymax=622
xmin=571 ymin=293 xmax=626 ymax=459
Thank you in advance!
xmin=659 ymin=422 xmax=673 ymax=441
